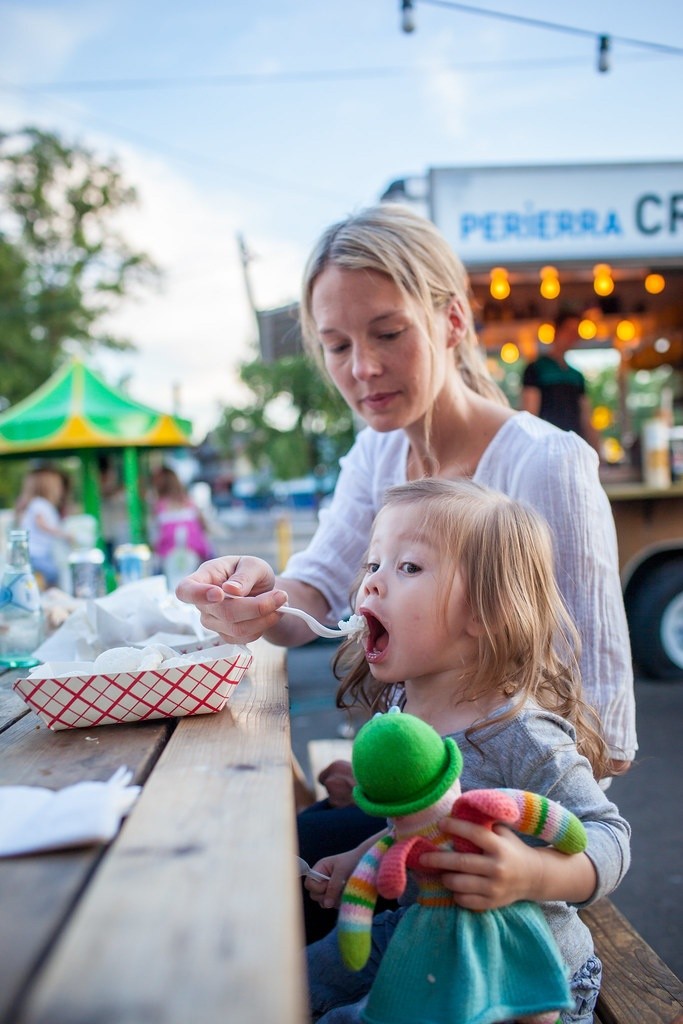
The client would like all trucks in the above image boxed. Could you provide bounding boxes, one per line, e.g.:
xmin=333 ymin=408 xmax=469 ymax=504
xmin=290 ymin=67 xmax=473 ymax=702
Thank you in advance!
xmin=383 ymin=160 xmax=683 ymax=679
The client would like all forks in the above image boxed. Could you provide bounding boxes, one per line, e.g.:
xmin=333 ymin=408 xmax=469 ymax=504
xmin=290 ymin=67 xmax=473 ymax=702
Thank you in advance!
xmin=225 ymin=593 xmax=360 ymax=637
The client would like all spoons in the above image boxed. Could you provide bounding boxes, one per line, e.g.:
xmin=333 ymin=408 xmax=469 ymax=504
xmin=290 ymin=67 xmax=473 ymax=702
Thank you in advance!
xmin=298 ymin=856 xmax=331 ymax=882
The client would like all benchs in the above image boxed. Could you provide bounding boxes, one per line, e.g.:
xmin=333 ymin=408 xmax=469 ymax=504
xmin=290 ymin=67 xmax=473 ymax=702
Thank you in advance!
xmin=304 ymin=740 xmax=683 ymax=1023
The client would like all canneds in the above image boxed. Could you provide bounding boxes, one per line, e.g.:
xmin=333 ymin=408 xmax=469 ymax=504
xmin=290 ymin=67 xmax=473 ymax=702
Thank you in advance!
xmin=113 ymin=543 xmax=154 ymax=586
xmin=66 ymin=548 xmax=105 ymax=599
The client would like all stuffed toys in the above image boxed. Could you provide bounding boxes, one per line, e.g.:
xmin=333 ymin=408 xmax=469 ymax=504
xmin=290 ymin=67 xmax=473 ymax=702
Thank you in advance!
xmin=333 ymin=711 xmax=588 ymax=1024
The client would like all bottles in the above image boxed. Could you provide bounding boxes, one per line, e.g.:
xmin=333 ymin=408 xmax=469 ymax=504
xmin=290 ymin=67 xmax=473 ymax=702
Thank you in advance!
xmin=0 ymin=531 xmax=40 ymax=666
xmin=642 ymin=419 xmax=671 ymax=489
xmin=158 ymin=509 xmax=207 ymax=594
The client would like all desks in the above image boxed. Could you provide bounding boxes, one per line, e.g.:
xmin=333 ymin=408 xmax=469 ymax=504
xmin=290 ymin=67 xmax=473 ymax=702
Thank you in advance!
xmin=0 ymin=620 xmax=317 ymax=1024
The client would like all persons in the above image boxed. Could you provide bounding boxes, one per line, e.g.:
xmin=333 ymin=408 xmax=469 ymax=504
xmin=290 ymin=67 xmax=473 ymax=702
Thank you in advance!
xmin=305 ymin=477 xmax=634 ymax=1024
xmin=13 ymin=467 xmax=101 ymax=592
xmin=173 ymin=205 xmax=640 ymax=947
xmin=147 ymin=465 xmax=217 ymax=581
xmin=520 ymin=311 xmax=603 ymax=461
